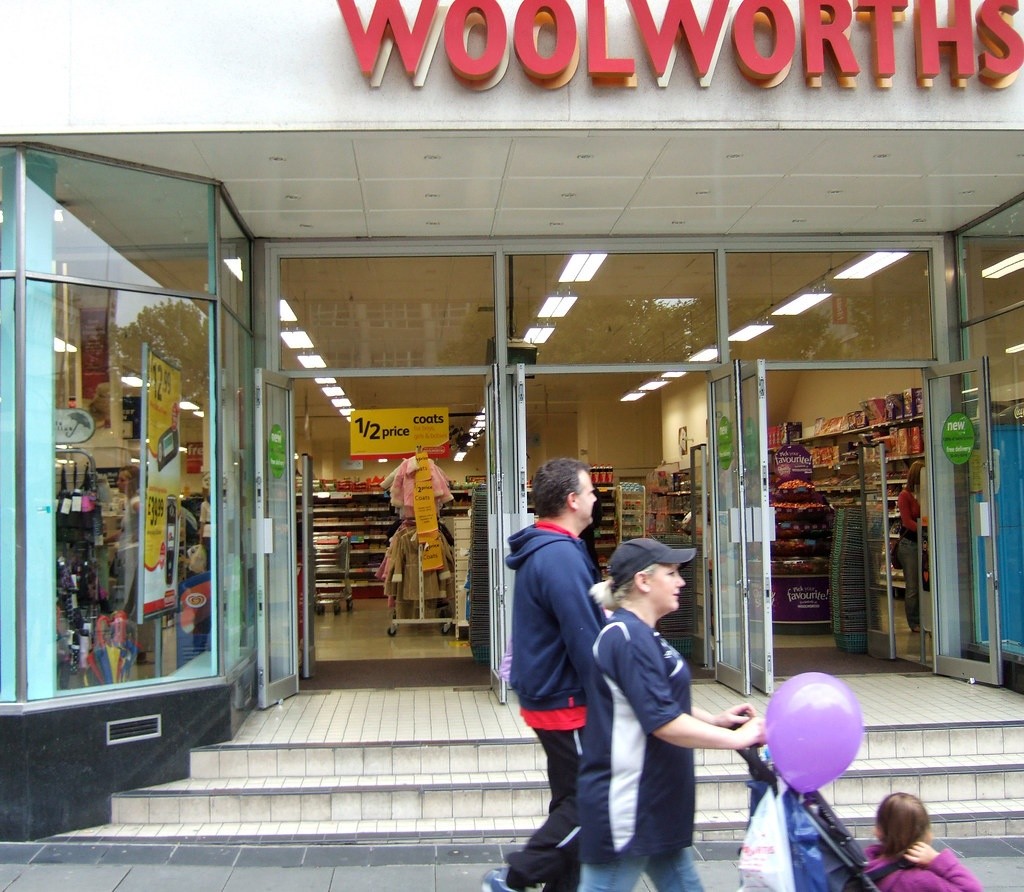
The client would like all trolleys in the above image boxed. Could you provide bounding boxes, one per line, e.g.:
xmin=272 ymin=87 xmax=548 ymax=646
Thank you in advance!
xmin=313 ymin=531 xmax=354 ymax=614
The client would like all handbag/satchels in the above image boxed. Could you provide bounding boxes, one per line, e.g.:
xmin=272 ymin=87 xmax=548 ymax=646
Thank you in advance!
xmin=738 ymin=777 xmax=795 ymax=892
xmin=187 ymin=544 xmax=207 ymax=573
xmin=891 ymin=543 xmax=903 ymax=570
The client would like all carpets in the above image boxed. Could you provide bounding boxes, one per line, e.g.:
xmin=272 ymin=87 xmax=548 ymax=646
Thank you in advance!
xmin=299 ymin=646 xmax=933 ymax=690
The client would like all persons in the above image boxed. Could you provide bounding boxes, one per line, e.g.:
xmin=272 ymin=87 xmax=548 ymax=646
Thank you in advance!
xmin=101 ymin=464 xmax=140 ymax=616
xmin=898 ymin=460 xmax=927 ymax=632
xmin=864 ymin=791 xmax=982 ymax=892
xmin=577 ymin=538 xmax=766 ymax=892
xmin=481 ymin=456 xmax=613 ymax=892
xmin=197 ymin=491 xmax=211 ymax=572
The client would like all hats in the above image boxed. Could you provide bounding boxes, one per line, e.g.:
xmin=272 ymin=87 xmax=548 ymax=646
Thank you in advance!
xmin=608 ymin=538 xmax=698 ymax=592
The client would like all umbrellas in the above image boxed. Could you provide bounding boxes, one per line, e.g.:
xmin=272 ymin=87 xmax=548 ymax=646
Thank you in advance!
xmin=87 ymin=609 xmax=139 ymax=684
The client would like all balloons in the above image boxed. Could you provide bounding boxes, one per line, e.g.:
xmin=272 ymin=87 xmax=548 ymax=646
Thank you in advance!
xmin=765 ymin=672 xmax=866 ymax=795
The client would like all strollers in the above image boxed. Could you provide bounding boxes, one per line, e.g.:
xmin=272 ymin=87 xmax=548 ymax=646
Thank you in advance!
xmin=729 ymin=711 xmax=881 ymax=892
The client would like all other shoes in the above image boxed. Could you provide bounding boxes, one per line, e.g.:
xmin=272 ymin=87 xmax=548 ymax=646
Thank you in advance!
xmin=481 ymin=867 xmax=540 ymax=892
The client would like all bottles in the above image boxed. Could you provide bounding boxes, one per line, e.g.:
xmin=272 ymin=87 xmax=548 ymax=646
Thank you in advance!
xmin=589 ymin=463 xmax=614 ymax=487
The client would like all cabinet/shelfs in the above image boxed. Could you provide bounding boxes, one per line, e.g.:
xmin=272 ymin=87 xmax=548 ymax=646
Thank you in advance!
xmin=597 ymin=482 xmax=646 ymax=581
xmin=746 ymin=445 xmax=832 ymax=625
xmin=768 ymin=415 xmax=928 ymax=599
xmin=296 ymin=489 xmax=535 ymax=599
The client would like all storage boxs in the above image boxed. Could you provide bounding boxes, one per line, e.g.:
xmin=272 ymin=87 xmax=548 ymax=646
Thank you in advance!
xmin=898 ymin=427 xmax=910 ymax=455
xmin=910 ymin=426 xmax=923 ymax=454
xmin=768 ymin=420 xmax=802 ymax=447
xmin=827 ymin=506 xmax=884 ymax=653
xmin=468 ymin=485 xmax=493 ymax=666
xmin=832 ymin=445 xmax=847 ymax=463
xmin=646 ymin=530 xmax=702 ymax=659
xmin=903 ymin=387 xmax=922 ymax=419
